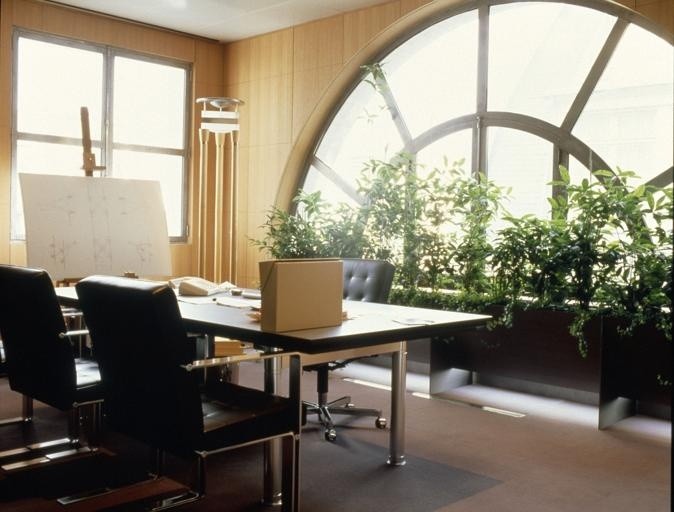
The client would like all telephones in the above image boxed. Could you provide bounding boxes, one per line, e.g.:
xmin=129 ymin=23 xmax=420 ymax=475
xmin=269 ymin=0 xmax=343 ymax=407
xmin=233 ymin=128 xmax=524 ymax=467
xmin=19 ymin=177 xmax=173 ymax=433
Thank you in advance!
xmin=179 ymin=276 xmax=219 ymax=296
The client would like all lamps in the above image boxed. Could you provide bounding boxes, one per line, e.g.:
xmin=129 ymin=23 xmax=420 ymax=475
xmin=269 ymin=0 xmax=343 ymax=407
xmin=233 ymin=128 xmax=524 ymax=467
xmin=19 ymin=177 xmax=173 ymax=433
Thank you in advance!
xmin=193 ymin=94 xmax=246 ymax=289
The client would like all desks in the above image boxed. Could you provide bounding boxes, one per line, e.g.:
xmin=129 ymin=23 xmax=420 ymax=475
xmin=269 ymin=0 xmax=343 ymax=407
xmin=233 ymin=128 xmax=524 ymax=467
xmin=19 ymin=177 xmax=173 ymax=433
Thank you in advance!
xmin=47 ymin=274 xmax=495 ymax=511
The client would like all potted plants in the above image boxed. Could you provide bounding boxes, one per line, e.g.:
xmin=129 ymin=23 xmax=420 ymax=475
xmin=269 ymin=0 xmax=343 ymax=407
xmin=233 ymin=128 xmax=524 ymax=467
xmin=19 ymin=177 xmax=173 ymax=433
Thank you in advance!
xmin=258 ymin=168 xmax=674 ymax=429
